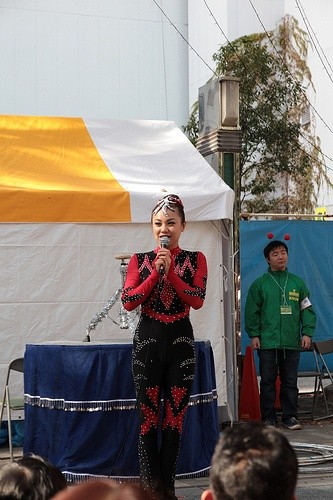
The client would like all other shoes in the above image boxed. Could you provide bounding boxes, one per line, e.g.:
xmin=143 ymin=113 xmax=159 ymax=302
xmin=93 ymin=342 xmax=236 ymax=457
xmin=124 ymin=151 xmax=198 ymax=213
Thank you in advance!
xmin=262 ymin=419 xmax=277 ymax=430
xmin=282 ymin=416 xmax=301 ymax=430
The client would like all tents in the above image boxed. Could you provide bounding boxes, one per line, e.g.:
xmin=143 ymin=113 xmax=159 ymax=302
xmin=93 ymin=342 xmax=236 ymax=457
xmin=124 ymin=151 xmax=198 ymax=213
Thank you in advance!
xmin=0 ymin=112 xmax=237 ymax=428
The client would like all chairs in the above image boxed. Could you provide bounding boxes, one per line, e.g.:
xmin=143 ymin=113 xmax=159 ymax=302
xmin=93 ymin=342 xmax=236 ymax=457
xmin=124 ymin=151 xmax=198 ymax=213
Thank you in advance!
xmin=0 ymin=358 xmax=24 ymax=463
xmin=275 ymin=339 xmax=333 ymax=422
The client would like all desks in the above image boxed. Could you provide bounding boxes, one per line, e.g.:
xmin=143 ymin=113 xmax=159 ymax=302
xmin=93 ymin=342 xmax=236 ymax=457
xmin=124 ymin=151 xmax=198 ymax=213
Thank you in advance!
xmin=23 ymin=341 xmax=220 ymax=485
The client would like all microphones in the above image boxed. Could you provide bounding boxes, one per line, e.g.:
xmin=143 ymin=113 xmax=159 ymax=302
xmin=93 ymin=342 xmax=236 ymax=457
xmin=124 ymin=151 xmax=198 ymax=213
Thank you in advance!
xmin=160 ymin=236 xmax=171 ymax=272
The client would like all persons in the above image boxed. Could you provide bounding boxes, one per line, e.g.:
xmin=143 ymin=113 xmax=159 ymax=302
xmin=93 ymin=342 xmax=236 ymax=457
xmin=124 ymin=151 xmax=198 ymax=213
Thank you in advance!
xmin=244 ymin=240 xmax=316 ymax=430
xmin=120 ymin=194 xmax=208 ymax=500
xmin=0 ymin=450 xmax=67 ymax=500
xmin=200 ymin=421 xmax=300 ymax=500
xmin=48 ymin=475 xmax=155 ymax=500
